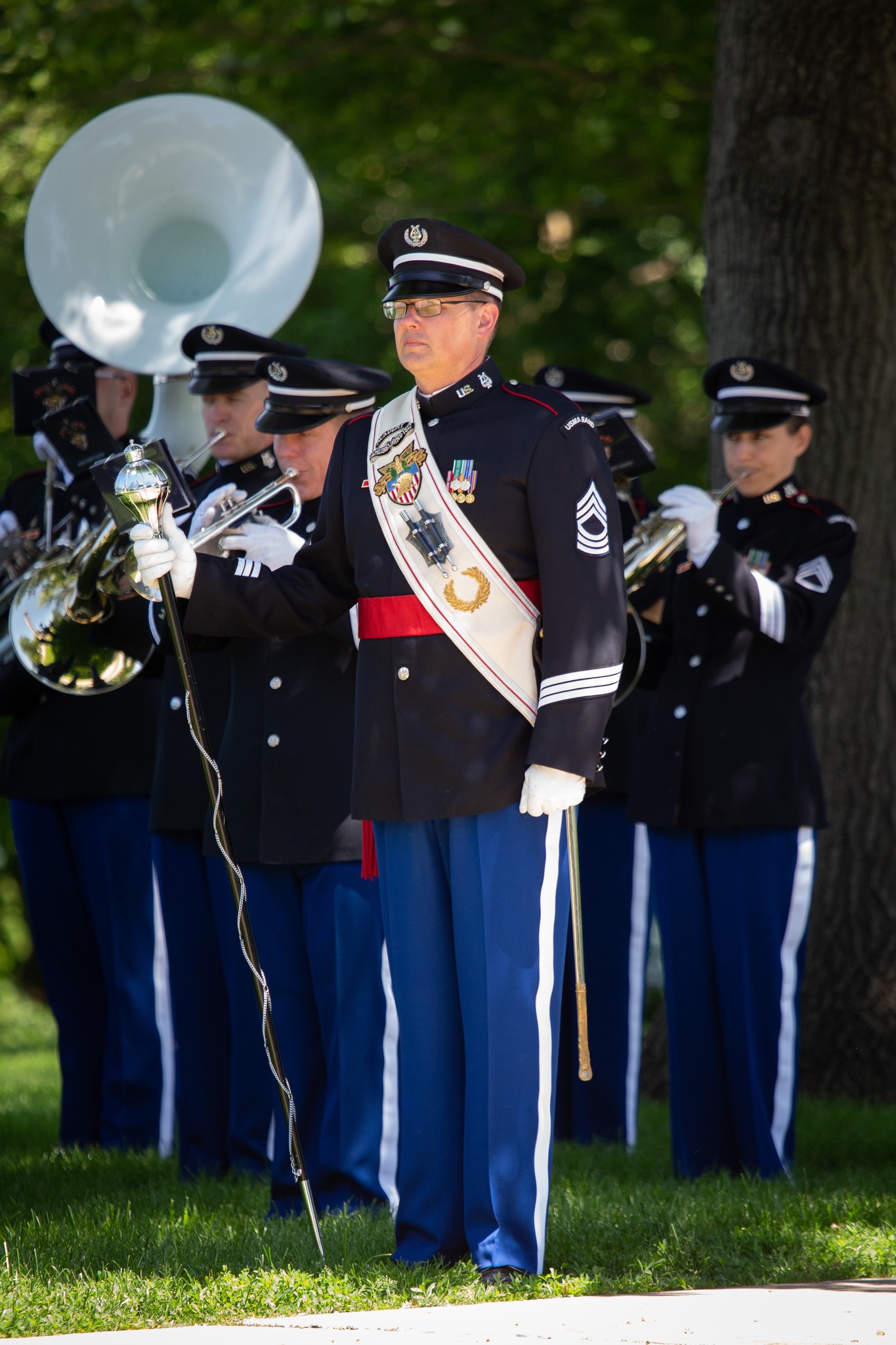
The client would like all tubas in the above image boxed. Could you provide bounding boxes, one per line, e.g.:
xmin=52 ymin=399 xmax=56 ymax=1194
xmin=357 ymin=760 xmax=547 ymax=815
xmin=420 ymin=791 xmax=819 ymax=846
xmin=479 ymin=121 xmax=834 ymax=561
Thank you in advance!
xmin=8 ymin=426 xmax=219 ymax=695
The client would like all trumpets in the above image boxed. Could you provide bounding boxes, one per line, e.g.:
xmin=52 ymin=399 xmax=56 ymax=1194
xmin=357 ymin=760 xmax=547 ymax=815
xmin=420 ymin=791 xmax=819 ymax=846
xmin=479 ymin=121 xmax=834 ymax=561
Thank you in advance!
xmin=124 ymin=463 xmax=303 ymax=610
xmin=620 ymin=462 xmax=766 ymax=597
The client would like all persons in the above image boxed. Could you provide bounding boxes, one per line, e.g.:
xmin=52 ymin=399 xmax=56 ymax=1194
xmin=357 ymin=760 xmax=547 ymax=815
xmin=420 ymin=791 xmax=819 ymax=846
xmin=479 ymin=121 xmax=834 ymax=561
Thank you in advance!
xmin=634 ymin=349 xmax=859 ymax=1184
xmin=0 ymin=318 xmax=662 ymax=1229
xmin=312 ymin=217 xmax=629 ymax=1286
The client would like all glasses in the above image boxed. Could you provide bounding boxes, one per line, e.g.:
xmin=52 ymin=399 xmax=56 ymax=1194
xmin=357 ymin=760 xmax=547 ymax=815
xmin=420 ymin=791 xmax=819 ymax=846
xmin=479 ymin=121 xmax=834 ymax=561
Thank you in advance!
xmin=380 ymin=300 xmax=487 ymax=320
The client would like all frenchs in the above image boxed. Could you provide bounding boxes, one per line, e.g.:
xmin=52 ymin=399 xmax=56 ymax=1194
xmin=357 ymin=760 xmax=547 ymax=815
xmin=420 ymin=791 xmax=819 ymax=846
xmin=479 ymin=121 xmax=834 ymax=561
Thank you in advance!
xmin=23 ymin=93 xmax=328 ymax=480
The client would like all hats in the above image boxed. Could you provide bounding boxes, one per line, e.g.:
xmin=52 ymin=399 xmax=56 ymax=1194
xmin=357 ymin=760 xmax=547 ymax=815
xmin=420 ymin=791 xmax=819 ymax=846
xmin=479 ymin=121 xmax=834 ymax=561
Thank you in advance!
xmin=534 ymin=364 xmax=652 ymax=419
xmin=377 ymin=218 xmax=525 ymax=310
xmin=179 ymin=323 xmax=310 ymax=395
xmin=701 ymin=357 xmax=828 ymax=435
xmin=254 ymin=356 xmax=391 ymax=435
xmin=38 ymin=315 xmax=109 ymax=371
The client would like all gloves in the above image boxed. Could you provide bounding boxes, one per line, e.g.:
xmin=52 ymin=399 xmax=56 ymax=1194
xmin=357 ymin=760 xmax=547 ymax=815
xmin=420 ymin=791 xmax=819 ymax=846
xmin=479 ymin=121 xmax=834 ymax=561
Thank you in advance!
xmin=130 ymin=501 xmax=200 ymax=600
xmin=186 ymin=483 xmax=247 ymax=558
xmin=658 ymin=484 xmax=719 ymax=569
xmin=34 ymin=432 xmax=76 ymax=488
xmin=518 ymin=763 xmax=586 ymax=816
xmin=1 ymin=511 xmax=19 ymax=538
xmin=218 ymin=516 xmax=307 ymax=571
